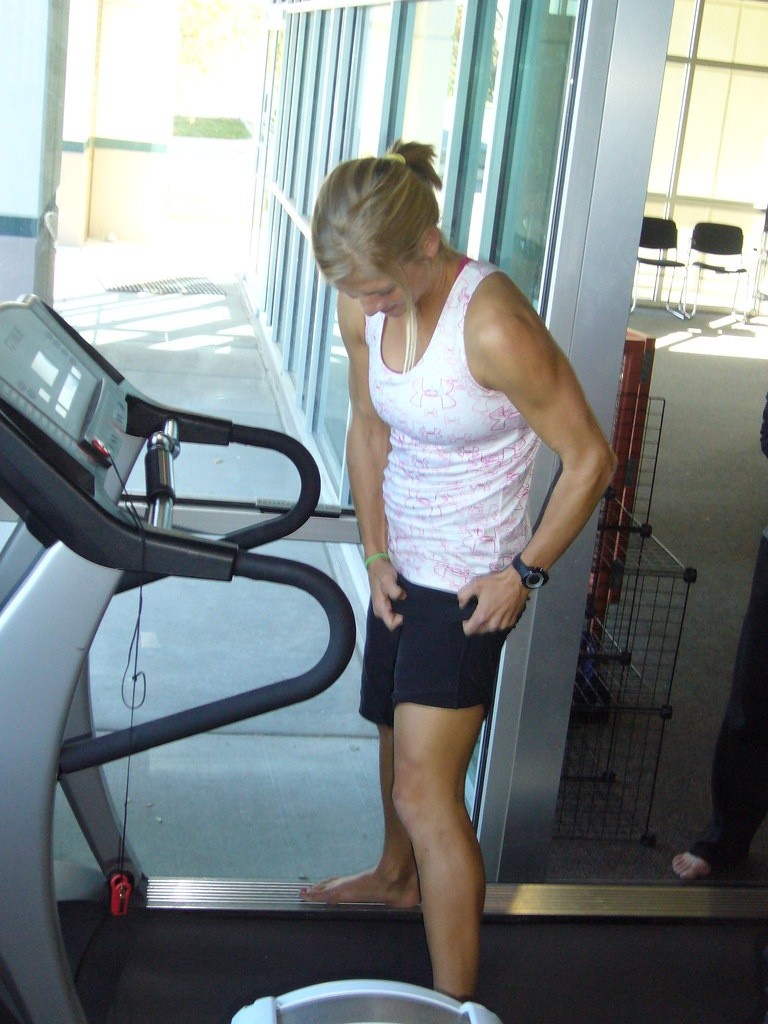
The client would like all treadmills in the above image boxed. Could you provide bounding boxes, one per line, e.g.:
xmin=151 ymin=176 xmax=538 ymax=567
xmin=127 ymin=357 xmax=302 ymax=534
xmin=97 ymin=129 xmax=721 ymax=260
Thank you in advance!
xmin=0 ymin=296 xmax=768 ymax=1024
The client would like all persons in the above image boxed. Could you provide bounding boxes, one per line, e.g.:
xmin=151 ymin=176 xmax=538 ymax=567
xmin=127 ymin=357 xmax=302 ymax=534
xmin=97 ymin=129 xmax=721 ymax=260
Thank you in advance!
xmin=671 ymin=380 xmax=767 ymax=882
xmin=299 ymin=140 xmax=620 ymax=1003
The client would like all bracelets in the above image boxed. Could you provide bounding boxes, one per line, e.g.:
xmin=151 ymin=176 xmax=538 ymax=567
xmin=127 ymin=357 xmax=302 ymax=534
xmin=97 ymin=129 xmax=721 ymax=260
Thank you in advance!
xmin=365 ymin=553 xmax=389 ymax=569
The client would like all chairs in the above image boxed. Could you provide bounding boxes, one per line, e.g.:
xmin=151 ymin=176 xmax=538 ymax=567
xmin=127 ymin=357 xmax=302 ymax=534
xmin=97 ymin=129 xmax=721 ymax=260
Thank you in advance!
xmin=629 ymin=217 xmax=688 ymax=321
xmin=677 ymin=222 xmax=751 ymax=324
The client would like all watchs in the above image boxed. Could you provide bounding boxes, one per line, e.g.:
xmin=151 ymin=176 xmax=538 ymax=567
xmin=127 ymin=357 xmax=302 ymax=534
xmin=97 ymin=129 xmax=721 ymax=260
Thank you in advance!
xmin=512 ymin=552 xmax=550 ymax=590
